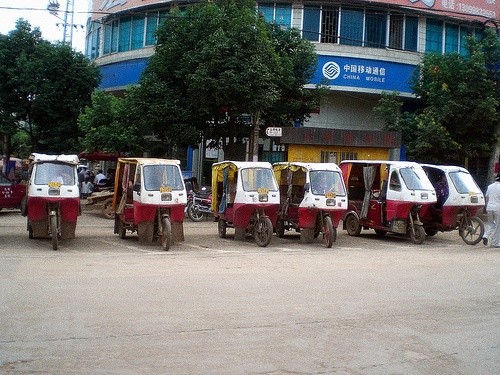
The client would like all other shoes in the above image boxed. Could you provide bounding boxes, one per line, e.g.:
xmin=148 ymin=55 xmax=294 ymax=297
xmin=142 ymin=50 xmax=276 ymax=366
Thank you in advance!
xmin=483 ymin=237 xmax=488 ymax=245
xmin=494 ymin=245 xmax=500 ymax=248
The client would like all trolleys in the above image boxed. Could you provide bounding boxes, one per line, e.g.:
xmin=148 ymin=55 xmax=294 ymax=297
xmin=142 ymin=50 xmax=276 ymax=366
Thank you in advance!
xmin=81 ymin=192 xmax=116 ymax=219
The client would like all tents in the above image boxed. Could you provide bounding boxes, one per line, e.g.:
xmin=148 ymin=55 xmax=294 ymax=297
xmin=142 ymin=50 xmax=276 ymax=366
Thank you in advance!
xmin=79 ymin=145 xmax=119 ymax=161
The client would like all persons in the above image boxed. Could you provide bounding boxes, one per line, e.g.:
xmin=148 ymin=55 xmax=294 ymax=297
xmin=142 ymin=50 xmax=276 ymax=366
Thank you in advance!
xmin=483 ymin=174 xmax=500 ymax=248
xmin=435 ymin=172 xmax=449 ymax=207
xmin=312 ymin=173 xmax=336 ymax=196
xmin=76 ymin=167 xmax=115 ymax=198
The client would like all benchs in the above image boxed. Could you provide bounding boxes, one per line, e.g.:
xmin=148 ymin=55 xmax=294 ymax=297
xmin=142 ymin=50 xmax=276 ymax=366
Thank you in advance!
xmin=350 ymin=180 xmax=376 ymax=209
xmin=281 ymin=184 xmax=305 ymax=215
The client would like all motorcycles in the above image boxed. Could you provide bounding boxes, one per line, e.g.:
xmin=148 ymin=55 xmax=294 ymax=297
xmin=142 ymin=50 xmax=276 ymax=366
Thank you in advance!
xmin=188 ymin=187 xmax=215 ymax=221
xmin=113 ymin=157 xmax=187 ymax=251
xmin=212 ymin=160 xmax=281 ymax=247
xmin=273 ymin=161 xmax=348 ymax=248
xmin=22 ymin=152 xmax=82 ymax=251
xmin=339 ymin=160 xmax=437 ymax=245
xmin=421 ymin=164 xmax=486 ymax=245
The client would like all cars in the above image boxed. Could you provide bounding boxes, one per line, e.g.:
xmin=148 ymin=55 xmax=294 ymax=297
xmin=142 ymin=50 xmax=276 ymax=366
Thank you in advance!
xmin=0 ymin=173 xmax=28 ymax=212
